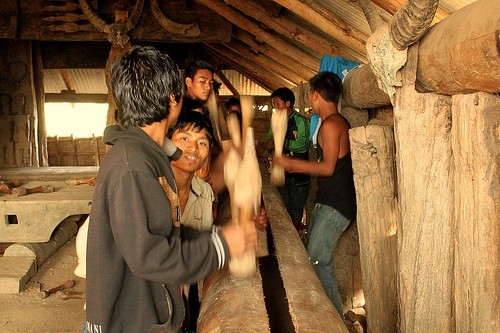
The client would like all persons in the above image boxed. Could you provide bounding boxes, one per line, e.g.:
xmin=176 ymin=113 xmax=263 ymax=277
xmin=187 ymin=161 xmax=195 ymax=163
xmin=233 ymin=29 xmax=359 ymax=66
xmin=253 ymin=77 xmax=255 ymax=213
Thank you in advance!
xmin=275 ymin=72 xmax=357 ymax=321
xmin=86 ymin=47 xmax=257 ymax=332
xmin=213 ymin=79 xmax=242 ymax=122
xmin=175 ymin=60 xmax=214 ymax=145
xmin=154 ymin=111 xmax=267 ymax=333
xmin=265 ymin=86 xmax=310 ymax=230
